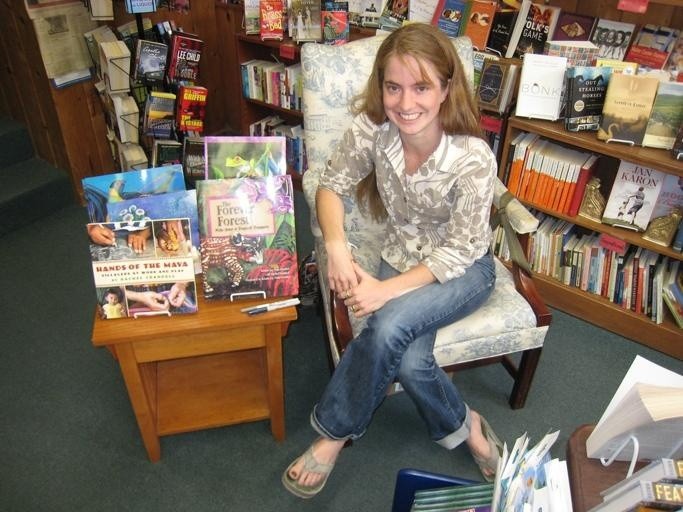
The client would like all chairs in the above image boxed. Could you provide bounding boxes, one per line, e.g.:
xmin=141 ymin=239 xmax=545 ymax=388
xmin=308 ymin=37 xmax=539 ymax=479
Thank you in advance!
xmin=298 ymin=31 xmax=552 ymax=447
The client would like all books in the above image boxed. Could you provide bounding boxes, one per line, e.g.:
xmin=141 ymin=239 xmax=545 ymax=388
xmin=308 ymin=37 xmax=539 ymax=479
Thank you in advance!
xmin=411 ymin=480 xmax=497 ymax=512
xmin=583 ymin=354 xmax=681 ymax=469
xmin=599 ymin=457 xmax=682 ymax=503
xmin=587 ymin=479 xmax=683 ymax=511
xmin=78 ymin=2 xmax=683 ymax=323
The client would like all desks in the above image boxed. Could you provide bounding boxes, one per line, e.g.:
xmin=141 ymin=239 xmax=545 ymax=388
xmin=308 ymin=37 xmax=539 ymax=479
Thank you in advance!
xmin=90 ymin=273 xmax=298 ymax=462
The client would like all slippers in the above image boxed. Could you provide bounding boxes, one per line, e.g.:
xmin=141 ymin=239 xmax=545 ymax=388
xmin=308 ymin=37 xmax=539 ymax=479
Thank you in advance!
xmin=281 ymin=436 xmax=335 ymax=500
xmin=472 ymin=415 xmax=504 ymax=482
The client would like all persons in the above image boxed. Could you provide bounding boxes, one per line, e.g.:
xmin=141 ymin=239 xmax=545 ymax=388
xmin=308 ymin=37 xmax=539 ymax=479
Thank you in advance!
xmin=279 ymin=25 xmax=508 ymax=498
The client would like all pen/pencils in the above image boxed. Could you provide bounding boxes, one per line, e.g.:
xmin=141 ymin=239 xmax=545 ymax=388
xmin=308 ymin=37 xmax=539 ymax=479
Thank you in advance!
xmin=99 ymin=224 xmax=118 ymax=247
xmin=241 ymin=298 xmax=301 ymax=315
